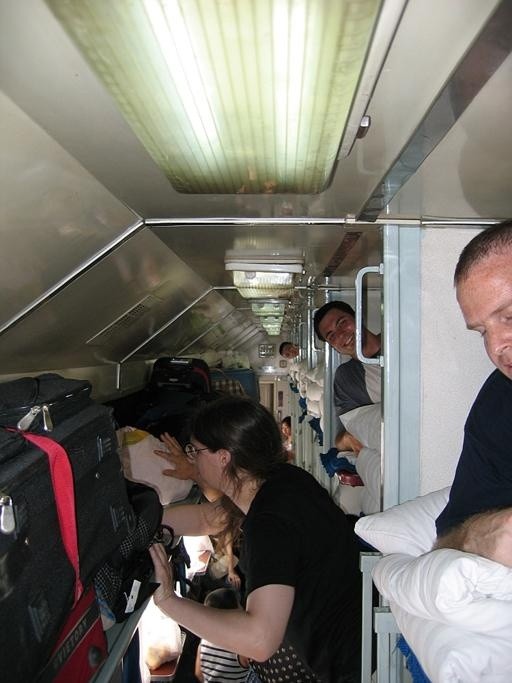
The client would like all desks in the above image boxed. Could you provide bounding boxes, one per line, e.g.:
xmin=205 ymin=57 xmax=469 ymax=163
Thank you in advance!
xmin=195 ymin=550 xmax=212 ymax=577
xmin=150 ymin=632 xmax=187 ymax=683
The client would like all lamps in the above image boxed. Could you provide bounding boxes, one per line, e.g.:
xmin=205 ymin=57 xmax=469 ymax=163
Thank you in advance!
xmin=46 ymin=1 xmax=410 ymax=194
xmin=226 ymin=258 xmax=308 ymax=336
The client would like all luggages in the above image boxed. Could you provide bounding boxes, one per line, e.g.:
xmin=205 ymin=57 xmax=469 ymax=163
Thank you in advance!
xmin=147 ymin=356 xmax=212 ymax=398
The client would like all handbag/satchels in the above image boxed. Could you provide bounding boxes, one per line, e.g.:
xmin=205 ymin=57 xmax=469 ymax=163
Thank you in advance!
xmin=204 ymin=347 xmax=264 ymax=402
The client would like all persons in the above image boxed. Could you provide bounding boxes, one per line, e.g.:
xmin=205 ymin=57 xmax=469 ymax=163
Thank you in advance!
xmin=138 ymin=394 xmax=379 ymax=683
xmin=429 ymin=216 xmax=512 ymax=572
xmin=313 ymin=300 xmax=382 ymax=456
xmin=278 ymin=340 xmax=301 ymax=360
xmin=207 ymin=528 xmax=243 ymax=589
xmin=152 ymin=429 xmax=229 ymax=503
xmin=194 ymin=587 xmax=251 ymax=682
xmin=277 ymin=415 xmax=293 ymax=455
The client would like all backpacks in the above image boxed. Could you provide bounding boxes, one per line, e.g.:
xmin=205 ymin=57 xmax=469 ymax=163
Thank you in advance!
xmin=1 ymin=374 xmax=195 ymax=680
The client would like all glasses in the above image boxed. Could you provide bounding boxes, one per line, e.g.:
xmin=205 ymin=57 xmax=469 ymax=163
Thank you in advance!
xmin=184 ymin=442 xmax=210 ymax=457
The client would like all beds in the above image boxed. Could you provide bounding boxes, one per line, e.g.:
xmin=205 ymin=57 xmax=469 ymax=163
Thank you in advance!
xmin=289 ymin=224 xmax=512 ymax=683
